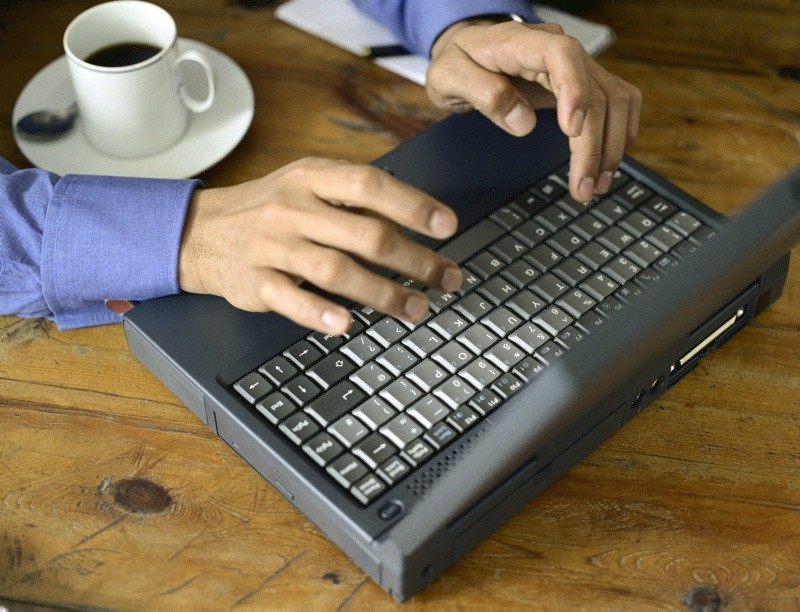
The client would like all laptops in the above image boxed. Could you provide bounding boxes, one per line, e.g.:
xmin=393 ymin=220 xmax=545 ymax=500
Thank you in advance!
xmin=120 ymin=105 xmax=800 ymax=606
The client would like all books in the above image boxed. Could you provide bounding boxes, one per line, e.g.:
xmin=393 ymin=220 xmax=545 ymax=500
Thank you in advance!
xmin=272 ymin=0 xmax=611 ymax=87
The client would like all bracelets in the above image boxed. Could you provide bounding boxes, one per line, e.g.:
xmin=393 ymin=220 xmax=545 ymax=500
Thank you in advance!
xmin=464 ymin=13 xmax=525 ymax=23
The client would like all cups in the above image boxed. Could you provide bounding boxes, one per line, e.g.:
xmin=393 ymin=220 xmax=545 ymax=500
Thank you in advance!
xmin=63 ymin=0 xmax=218 ymax=160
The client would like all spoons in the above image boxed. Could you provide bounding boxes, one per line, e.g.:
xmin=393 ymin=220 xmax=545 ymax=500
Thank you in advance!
xmin=17 ymin=100 xmax=79 ymax=142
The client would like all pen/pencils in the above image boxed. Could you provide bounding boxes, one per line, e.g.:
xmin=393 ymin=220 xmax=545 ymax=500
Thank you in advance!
xmin=363 ymin=44 xmax=410 ymax=58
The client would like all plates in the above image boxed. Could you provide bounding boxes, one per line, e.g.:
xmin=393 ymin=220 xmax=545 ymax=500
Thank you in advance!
xmin=11 ymin=36 xmax=257 ymax=180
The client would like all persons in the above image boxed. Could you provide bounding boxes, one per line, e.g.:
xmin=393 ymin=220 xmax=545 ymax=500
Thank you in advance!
xmin=0 ymin=0 xmax=641 ymax=335
